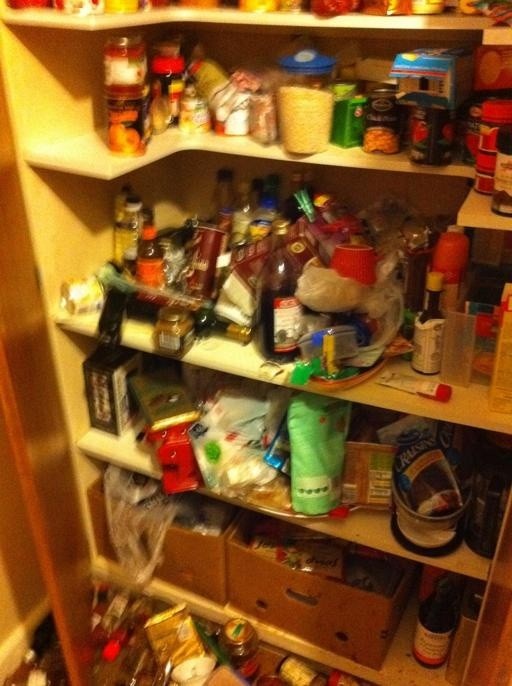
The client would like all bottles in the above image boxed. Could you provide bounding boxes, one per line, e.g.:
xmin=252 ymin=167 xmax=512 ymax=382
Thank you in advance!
xmin=109 ymin=180 xmax=166 ymax=293
xmin=252 ymin=221 xmax=302 ymax=364
xmin=276 ymin=48 xmax=336 ymax=156
xmin=430 ymin=220 xmax=471 ymax=313
xmin=413 ymin=271 xmax=447 ymax=375
xmin=23 ymin=583 xmax=156 ymax=686
xmin=411 ymin=573 xmax=457 ymax=670
xmin=333 ymin=311 xmax=380 ymax=353
xmin=274 ymin=653 xmax=330 ymax=686
xmin=201 ymin=167 xmax=310 ymax=246
xmin=125 ymin=297 xmax=255 ymax=346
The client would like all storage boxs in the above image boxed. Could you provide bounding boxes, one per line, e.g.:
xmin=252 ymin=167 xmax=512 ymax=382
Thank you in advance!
xmin=86 ymin=479 xmax=227 ymax=610
xmin=228 ymin=514 xmax=411 ymax=668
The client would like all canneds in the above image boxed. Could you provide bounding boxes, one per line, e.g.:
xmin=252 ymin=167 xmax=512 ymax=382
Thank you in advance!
xmin=406 ymin=106 xmax=455 ymax=166
xmin=185 ymin=225 xmax=224 ymax=300
xmin=363 ymin=87 xmax=402 ymax=155
xmin=457 ymin=93 xmax=497 ymax=166
xmin=104 ymin=94 xmax=148 ymax=158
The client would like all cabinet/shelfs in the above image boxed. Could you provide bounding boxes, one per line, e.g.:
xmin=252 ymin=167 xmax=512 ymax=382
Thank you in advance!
xmin=0 ymin=11 xmax=512 ymax=686
